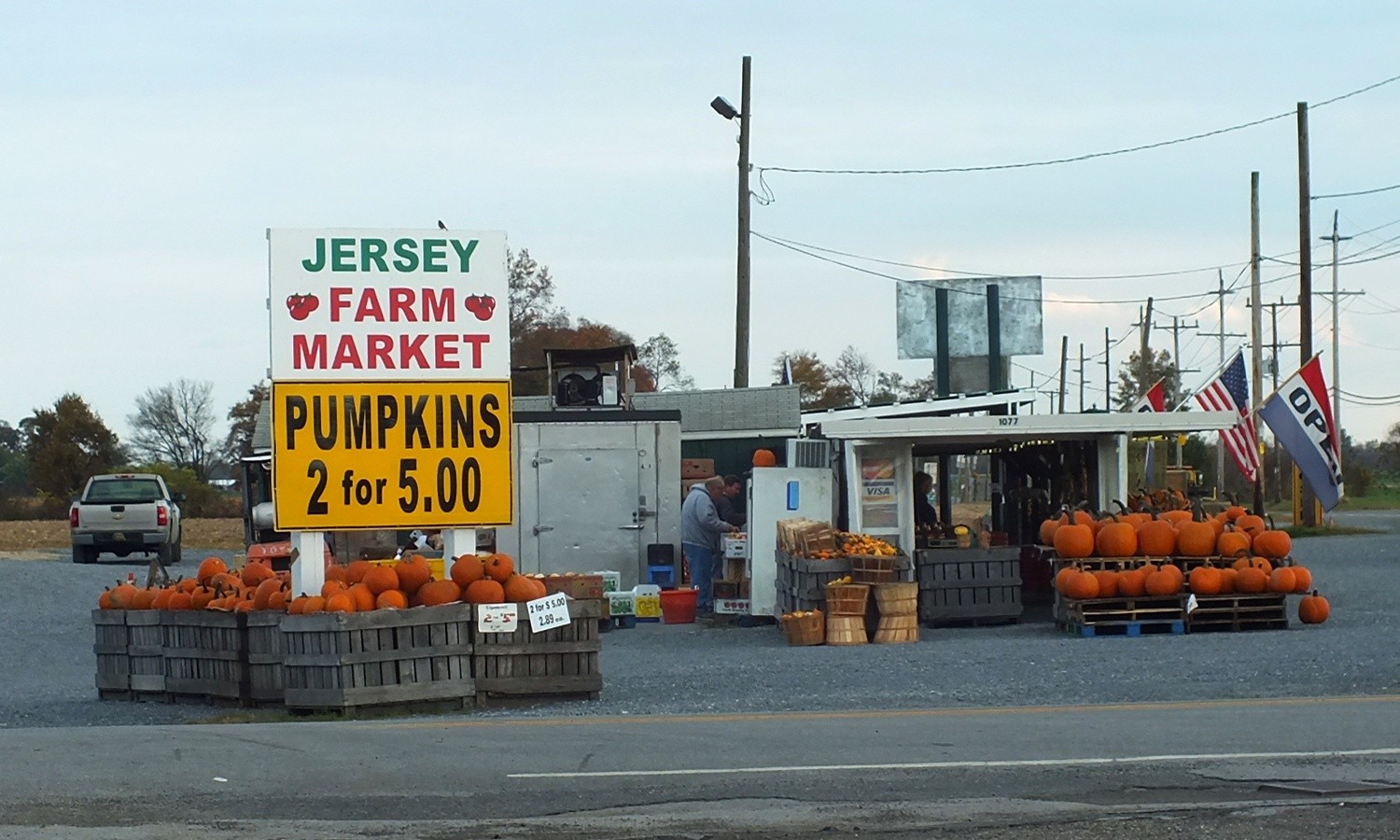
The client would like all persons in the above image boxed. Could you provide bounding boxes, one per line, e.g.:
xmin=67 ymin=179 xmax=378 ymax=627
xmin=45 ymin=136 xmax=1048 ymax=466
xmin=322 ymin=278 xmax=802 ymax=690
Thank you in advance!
xmin=681 ymin=475 xmax=740 ymax=615
xmin=711 ymin=477 xmax=746 ymax=580
xmin=913 ymin=472 xmax=937 ymax=524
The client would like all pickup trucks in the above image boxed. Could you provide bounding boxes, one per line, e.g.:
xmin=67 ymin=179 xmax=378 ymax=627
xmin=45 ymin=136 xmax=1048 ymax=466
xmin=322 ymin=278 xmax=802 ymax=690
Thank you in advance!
xmin=69 ymin=473 xmax=186 ymax=565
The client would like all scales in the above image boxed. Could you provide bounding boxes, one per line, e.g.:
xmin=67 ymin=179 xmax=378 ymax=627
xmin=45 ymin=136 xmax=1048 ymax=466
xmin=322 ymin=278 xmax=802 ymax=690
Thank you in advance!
xmin=927 ymin=539 xmax=958 ymax=547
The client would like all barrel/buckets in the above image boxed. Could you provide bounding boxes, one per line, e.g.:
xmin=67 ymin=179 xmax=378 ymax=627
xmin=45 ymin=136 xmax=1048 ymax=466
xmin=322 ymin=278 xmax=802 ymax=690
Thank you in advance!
xmin=782 ymin=556 xmax=920 ymax=645
xmin=658 ymin=591 xmax=697 ymax=624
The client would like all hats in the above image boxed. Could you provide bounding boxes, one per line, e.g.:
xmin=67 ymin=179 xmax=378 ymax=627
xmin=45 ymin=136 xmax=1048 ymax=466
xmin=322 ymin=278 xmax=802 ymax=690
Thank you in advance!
xmin=705 ymin=476 xmax=724 ymax=489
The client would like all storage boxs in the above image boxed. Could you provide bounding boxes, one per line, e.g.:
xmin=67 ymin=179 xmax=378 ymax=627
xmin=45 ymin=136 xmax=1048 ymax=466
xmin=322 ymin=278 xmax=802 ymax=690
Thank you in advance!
xmin=546 ymin=570 xmax=662 ymax=617
xmin=681 ymin=458 xmax=716 ymax=499
xmin=713 ymin=530 xmax=751 ymax=614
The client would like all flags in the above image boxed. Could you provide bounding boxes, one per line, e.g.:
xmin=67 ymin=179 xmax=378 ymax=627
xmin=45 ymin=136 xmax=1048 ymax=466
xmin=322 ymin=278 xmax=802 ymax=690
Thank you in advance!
xmin=1196 ymin=350 xmax=1260 ymax=484
xmin=1131 ymin=382 xmax=1163 ymax=413
xmin=1258 ymin=357 xmax=1344 ymax=513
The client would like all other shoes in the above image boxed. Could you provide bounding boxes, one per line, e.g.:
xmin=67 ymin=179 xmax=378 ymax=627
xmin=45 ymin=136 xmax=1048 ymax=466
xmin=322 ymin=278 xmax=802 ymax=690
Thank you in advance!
xmin=696 ymin=610 xmax=713 ymax=618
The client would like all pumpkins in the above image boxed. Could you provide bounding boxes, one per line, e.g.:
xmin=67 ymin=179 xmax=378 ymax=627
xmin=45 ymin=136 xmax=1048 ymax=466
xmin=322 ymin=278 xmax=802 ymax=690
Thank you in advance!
xmin=289 ymin=554 xmax=549 ymax=613
xmin=99 ymin=556 xmax=293 ymax=611
xmin=1041 ymin=487 xmax=1312 ymax=600
xmin=753 ymin=450 xmax=776 ymax=466
xmin=1299 ymin=590 xmax=1329 ymax=624
xmin=780 ymin=532 xmax=897 ymax=618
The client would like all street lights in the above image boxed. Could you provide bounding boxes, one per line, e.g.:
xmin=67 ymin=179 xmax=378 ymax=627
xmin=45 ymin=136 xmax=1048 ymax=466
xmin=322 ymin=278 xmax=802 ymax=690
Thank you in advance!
xmin=711 ymin=94 xmax=752 ymax=387
